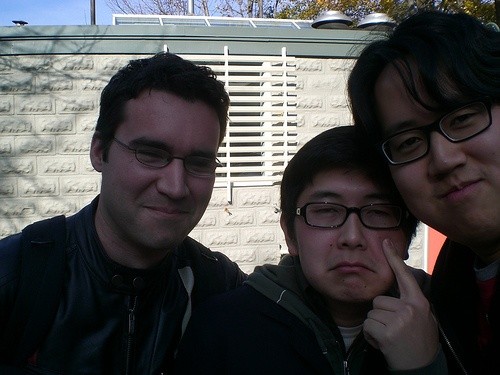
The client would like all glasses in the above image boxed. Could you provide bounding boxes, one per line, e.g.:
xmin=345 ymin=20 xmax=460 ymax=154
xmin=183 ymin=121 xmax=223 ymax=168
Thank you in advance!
xmin=289 ymin=201 xmax=410 ymax=231
xmin=379 ymin=95 xmax=500 ymax=167
xmin=111 ymin=136 xmax=224 ymax=177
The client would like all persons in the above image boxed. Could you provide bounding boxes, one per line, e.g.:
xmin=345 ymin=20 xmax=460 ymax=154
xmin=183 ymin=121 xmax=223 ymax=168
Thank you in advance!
xmin=0 ymin=51 xmax=247 ymax=375
xmin=344 ymin=5 xmax=500 ymax=375
xmin=229 ymin=126 xmax=447 ymax=375
